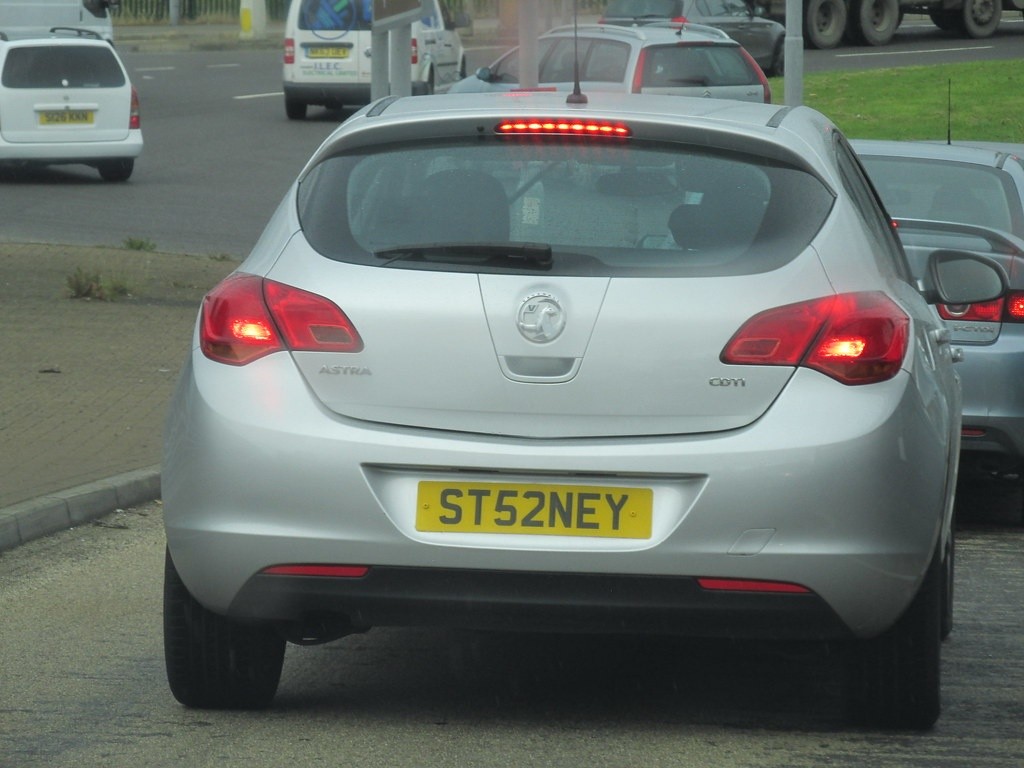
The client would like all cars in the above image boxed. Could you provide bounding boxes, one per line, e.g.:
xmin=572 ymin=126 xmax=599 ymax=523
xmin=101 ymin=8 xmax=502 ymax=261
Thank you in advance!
xmin=755 ymin=137 xmax=1023 ymax=522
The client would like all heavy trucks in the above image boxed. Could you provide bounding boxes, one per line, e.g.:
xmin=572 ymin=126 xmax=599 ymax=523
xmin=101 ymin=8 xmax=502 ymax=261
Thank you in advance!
xmin=748 ymin=1 xmax=1023 ymax=51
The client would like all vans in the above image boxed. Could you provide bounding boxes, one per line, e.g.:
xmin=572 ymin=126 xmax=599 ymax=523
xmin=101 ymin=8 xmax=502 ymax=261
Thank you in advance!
xmin=284 ymin=1 xmax=469 ymax=119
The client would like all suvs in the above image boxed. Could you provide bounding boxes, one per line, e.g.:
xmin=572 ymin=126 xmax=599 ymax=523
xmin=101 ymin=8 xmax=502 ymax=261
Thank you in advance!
xmin=0 ymin=0 xmax=118 ymax=51
xmin=0 ymin=38 xmax=142 ymax=183
xmin=160 ymin=84 xmax=1009 ymax=733
xmin=442 ymin=24 xmax=775 ymax=97
xmin=596 ymin=0 xmax=789 ymax=75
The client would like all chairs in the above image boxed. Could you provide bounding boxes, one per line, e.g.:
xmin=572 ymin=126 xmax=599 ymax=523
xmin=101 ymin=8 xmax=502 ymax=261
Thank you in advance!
xmin=668 ymin=202 xmax=775 ymax=251
xmin=402 ymin=170 xmax=509 ymax=243
